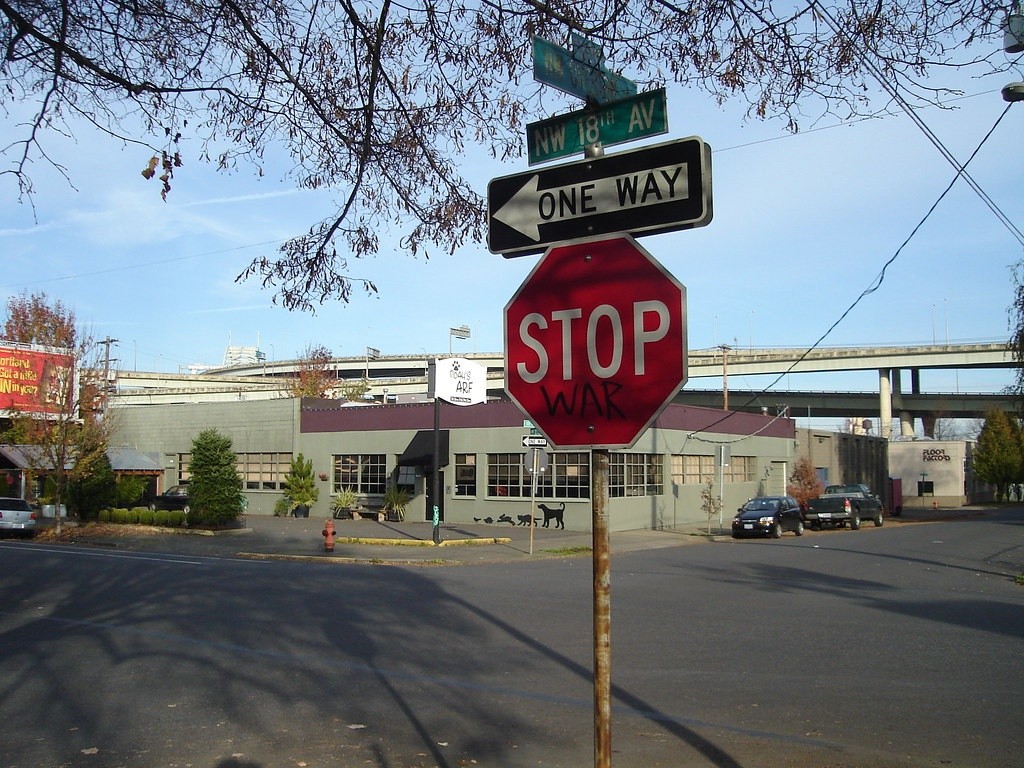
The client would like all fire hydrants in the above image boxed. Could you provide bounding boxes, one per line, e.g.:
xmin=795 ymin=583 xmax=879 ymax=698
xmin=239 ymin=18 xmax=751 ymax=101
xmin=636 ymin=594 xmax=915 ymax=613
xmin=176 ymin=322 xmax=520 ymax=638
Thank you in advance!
xmin=322 ymin=517 xmax=336 ymax=553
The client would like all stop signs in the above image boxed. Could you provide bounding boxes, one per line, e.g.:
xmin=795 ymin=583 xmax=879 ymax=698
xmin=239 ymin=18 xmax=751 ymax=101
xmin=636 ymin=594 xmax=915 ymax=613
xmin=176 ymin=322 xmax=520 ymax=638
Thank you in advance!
xmin=502 ymin=233 xmax=688 ymax=449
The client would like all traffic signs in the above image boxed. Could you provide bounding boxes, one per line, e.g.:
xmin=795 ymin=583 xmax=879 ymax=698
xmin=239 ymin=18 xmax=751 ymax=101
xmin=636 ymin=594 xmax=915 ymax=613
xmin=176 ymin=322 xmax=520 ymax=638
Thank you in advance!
xmin=521 ymin=434 xmax=548 ymax=449
xmin=487 ymin=135 xmax=712 ymax=254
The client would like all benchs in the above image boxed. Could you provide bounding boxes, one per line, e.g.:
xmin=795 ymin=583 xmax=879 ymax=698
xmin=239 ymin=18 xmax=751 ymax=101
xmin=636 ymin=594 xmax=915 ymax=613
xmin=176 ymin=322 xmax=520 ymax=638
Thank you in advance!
xmin=349 ymin=501 xmax=390 ymax=521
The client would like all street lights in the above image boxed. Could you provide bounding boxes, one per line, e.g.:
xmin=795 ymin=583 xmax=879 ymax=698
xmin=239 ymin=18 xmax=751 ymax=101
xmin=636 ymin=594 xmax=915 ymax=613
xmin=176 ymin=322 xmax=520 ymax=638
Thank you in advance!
xmin=132 ymin=339 xmax=136 ymax=372
xmin=944 ymin=298 xmax=950 ymax=346
xmin=713 ymin=314 xmax=718 ymax=348
xmin=421 ymin=347 xmax=426 ymax=378
xmin=336 ymin=344 xmax=343 ymax=380
xmin=750 ymin=310 xmax=756 ymax=349
xmin=269 ymin=344 xmax=274 ymax=377
xmin=931 ymin=303 xmax=937 ymax=345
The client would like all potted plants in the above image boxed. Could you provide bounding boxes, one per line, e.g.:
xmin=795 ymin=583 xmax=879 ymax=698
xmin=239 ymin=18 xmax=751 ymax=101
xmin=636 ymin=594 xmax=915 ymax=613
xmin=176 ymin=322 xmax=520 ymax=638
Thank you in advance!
xmin=274 ymin=497 xmax=290 ymax=518
xmin=329 ymin=483 xmax=360 ymax=519
xmin=284 ymin=453 xmax=320 ymax=518
xmin=375 ymin=480 xmax=418 ymax=524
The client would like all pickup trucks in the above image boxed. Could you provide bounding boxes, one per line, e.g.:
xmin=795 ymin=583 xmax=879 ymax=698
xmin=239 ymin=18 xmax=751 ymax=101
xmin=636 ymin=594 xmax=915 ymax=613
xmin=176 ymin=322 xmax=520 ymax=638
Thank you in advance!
xmin=805 ymin=483 xmax=884 ymax=531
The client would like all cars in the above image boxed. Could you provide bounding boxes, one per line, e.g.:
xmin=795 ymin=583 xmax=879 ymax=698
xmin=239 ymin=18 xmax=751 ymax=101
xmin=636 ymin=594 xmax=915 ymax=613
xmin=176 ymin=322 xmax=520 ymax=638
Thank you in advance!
xmin=731 ymin=496 xmax=804 ymax=539
xmin=147 ymin=484 xmax=193 ymax=515
xmin=0 ymin=497 xmax=36 ymax=539
